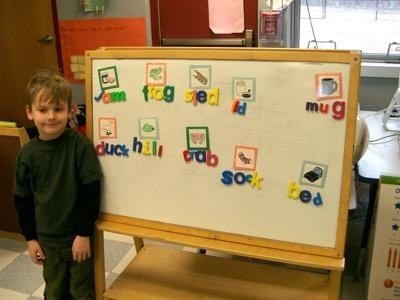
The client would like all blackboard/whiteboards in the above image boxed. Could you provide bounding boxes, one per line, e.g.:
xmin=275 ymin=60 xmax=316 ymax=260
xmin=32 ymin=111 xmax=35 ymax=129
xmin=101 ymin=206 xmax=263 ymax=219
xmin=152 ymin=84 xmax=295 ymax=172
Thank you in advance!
xmin=85 ymin=50 xmax=364 ymax=259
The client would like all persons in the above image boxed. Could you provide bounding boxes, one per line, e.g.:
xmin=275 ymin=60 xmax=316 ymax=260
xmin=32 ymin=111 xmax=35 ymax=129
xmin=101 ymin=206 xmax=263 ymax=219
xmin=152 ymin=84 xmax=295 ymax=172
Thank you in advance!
xmin=12 ymin=69 xmax=105 ymax=300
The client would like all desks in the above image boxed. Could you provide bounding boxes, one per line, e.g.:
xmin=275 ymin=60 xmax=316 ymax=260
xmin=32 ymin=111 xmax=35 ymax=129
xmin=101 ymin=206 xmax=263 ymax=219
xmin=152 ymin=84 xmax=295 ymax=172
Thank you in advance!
xmin=354 ymin=111 xmax=400 ymax=281
